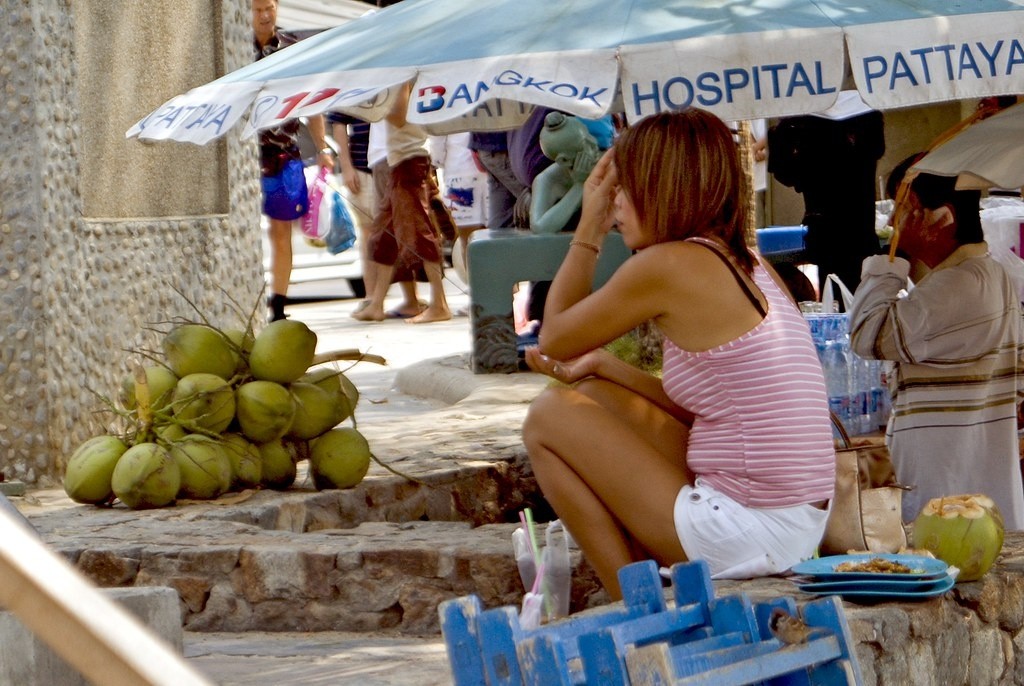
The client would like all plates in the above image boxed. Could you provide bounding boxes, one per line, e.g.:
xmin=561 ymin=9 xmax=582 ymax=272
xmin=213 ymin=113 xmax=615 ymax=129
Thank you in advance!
xmin=791 ymin=554 xmax=955 ymax=602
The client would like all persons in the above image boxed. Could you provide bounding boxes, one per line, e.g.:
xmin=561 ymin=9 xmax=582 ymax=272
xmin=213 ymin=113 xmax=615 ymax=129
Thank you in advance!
xmin=748 ymin=120 xmax=768 ymax=221
xmin=251 ymin=0 xmax=621 ymax=347
xmin=521 ymin=107 xmax=838 ymax=604
xmin=850 ymin=151 xmax=1024 ymax=534
xmin=529 ymin=112 xmax=603 ymax=234
xmin=767 ymin=112 xmax=883 ymax=314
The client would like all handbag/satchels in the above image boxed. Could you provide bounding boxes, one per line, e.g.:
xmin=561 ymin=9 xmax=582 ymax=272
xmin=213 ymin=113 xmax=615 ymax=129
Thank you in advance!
xmin=326 ymin=191 xmax=357 ymax=254
xmin=298 ymin=166 xmax=334 ymax=239
xmin=819 ymin=409 xmax=912 ymax=556
xmin=512 ymin=519 xmax=571 ymax=617
xmin=802 ymin=273 xmax=915 ymax=438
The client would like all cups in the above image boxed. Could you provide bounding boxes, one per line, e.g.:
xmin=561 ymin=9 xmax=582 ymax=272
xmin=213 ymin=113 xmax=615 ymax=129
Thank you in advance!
xmin=520 ymin=554 xmax=571 ymax=619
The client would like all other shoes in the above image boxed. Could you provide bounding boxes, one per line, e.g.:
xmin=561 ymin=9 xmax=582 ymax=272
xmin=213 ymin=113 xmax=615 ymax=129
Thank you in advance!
xmin=351 ymin=301 xmax=371 ymax=317
xmin=431 ymin=197 xmax=459 ymax=240
xmin=418 ymin=299 xmax=428 ymax=313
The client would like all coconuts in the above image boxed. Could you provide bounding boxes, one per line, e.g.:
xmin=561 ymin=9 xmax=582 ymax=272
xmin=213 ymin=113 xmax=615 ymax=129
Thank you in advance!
xmin=912 ymin=494 xmax=1005 ymax=583
xmin=63 ymin=321 xmax=372 ymax=510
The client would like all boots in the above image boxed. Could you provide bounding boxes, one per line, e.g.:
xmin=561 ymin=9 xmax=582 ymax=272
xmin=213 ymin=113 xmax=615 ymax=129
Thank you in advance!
xmin=269 ymin=293 xmax=286 ymax=322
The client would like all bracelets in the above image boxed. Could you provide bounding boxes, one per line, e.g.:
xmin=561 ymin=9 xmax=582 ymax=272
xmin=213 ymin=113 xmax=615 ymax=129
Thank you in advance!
xmin=569 ymin=240 xmax=602 ymax=253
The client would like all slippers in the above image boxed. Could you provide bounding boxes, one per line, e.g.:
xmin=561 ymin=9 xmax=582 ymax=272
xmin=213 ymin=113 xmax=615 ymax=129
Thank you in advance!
xmin=385 ymin=310 xmax=416 ymax=318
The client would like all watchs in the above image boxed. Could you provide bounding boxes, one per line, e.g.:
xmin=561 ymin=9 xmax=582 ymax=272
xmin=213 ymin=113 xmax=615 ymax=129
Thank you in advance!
xmin=318 ymin=147 xmax=332 ymax=156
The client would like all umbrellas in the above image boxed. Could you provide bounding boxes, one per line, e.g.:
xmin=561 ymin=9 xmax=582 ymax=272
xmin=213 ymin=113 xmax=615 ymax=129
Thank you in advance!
xmin=125 ymin=0 xmax=1024 ymax=148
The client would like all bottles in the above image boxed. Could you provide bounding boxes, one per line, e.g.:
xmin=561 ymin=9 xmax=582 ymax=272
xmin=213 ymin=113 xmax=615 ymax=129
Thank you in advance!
xmin=804 ymin=314 xmax=850 ymax=343
xmin=814 ymin=343 xmax=891 ymax=438
